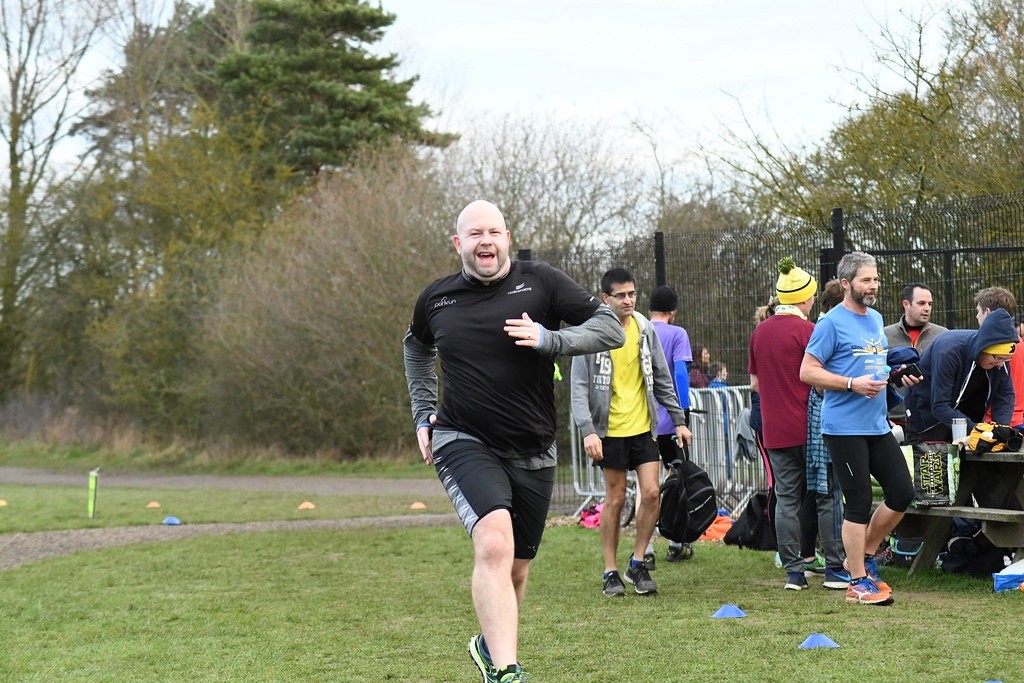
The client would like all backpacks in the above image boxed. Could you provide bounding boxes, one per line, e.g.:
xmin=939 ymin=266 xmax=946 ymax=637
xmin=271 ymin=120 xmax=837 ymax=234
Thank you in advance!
xmin=578 ymin=502 xmax=605 ymax=528
xmin=936 ymin=528 xmax=1009 ymax=577
xmin=622 ymin=438 xmax=719 ymax=543
xmin=724 ymin=495 xmax=778 ymax=551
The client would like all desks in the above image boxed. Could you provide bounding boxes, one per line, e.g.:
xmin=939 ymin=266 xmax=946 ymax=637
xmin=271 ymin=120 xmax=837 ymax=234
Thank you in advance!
xmin=906 ymin=449 xmax=1024 ymax=578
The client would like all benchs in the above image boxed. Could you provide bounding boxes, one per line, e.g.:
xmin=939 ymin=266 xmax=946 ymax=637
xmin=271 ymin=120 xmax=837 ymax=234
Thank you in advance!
xmin=868 ymin=501 xmax=1024 ymax=523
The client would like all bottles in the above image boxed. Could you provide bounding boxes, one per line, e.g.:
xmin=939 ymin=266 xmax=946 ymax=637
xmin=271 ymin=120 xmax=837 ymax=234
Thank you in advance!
xmin=864 ymin=366 xmax=892 ymax=399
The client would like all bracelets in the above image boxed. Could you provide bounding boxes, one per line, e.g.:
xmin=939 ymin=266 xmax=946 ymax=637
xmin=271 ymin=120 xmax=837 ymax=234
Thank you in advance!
xmin=675 ymin=424 xmax=686 ymax=428
xmin=847 ymin=377 xmax=853 ymax=393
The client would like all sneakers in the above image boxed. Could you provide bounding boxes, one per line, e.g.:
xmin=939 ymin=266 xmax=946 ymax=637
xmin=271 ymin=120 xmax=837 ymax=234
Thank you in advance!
xmin=804 ymin=558 xmax=826 ymax=577
xmin=784 ymin=571 xmax=808 ymax=591
xmin=666 ymin=543 xmax=694 ymax=562
xmin=865 ymin=560 xmax=892 ymax=593
xmin=602 ymin=570 xmax=625 ymax=598
xmin=468 ymin=633 xmax=522 ymax=683
xmin=844 ymin=577 xmax=893 ymax=605
xmin=824 ymin=569 xmax=851 ymax=589
xmin=629 ymin=551 xmax=655 ymax=571
xmin=624 ymin=554 xmax=657 ymax=594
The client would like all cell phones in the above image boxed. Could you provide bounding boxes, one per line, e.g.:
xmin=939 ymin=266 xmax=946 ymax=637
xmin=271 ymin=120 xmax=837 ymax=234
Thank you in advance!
xmin=891 ymin=363 xmax=922 ymax=388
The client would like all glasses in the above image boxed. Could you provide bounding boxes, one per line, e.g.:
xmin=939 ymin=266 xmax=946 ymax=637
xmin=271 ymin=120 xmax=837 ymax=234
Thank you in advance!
xmin=993 ymin=355 xmax=1013 ymax=363
xmin=607 ymin=292 xmax=637 ymax=300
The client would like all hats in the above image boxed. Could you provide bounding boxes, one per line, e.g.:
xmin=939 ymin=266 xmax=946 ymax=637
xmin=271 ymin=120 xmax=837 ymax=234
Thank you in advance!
xmin=649 ymin=286 xmax=679 ymax=312
xmin=982 ymin=341 xmax=1016 ymax=355
xmin=776 ymin=257 xmax=817 ymax=305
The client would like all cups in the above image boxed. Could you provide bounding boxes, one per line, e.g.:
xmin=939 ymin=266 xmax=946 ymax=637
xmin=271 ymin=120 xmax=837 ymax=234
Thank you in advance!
xmin=952 ymin=417 xmax=967 ymax=443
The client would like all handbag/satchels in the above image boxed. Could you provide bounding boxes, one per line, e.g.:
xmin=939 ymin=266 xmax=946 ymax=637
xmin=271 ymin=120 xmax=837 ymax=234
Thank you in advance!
xmin=888 ymin=535 xmax=925 ymax=568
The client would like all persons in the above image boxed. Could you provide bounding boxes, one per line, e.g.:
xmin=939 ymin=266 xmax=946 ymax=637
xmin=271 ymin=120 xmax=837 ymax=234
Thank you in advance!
xmin=973 ymin=286 xmax=1024 ymax=434
xmin=904 ymin=308 xmax=1020 ymax=445
xmin=748 ymin=251 xmax=924 ymax=605
xmin=570 ymin=267 xmax=693 ymax=599
xmin=401 ymin=200 xmax=626 ymax=682
xmin=688 ymin=345 xmax=735 ymax=494
xmin=883 ymin=282 xmax=949 ymax=412
